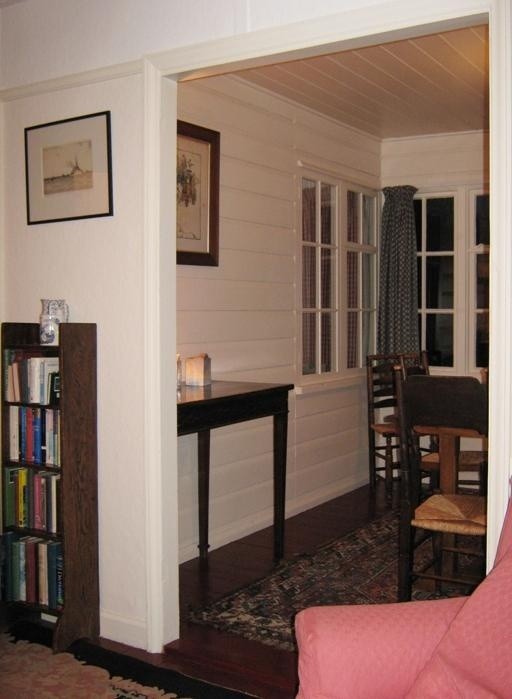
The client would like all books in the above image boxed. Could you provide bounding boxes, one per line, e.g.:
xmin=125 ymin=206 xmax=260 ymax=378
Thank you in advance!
xmin=0 ymin=348 xmax=64 ymax=608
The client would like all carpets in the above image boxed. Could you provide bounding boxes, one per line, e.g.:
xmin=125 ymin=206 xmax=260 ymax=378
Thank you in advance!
xmin=194 ymin=506 xmax=487 ymax=645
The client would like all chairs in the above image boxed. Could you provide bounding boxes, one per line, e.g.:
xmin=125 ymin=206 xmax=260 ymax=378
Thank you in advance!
xmin=295 ymin=490 xmax=511 ymax=699
xmin=365 ymin=348 xmax=488 ymax=601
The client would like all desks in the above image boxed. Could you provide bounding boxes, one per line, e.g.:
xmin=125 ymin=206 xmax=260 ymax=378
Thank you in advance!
xmin=177 ymin=376 xmax=293 ymax=566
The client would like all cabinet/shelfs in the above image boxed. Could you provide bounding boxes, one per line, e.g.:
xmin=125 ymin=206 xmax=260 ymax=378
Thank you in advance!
xmin=1 ymin=319 xmax=98 ymax=639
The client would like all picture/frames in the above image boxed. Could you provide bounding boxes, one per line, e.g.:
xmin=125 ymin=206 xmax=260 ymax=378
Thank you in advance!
xmin=178 ymin=118 xmax=221 ymax=268
xmin=23 ymin=111 xmax=113 ymax=226
